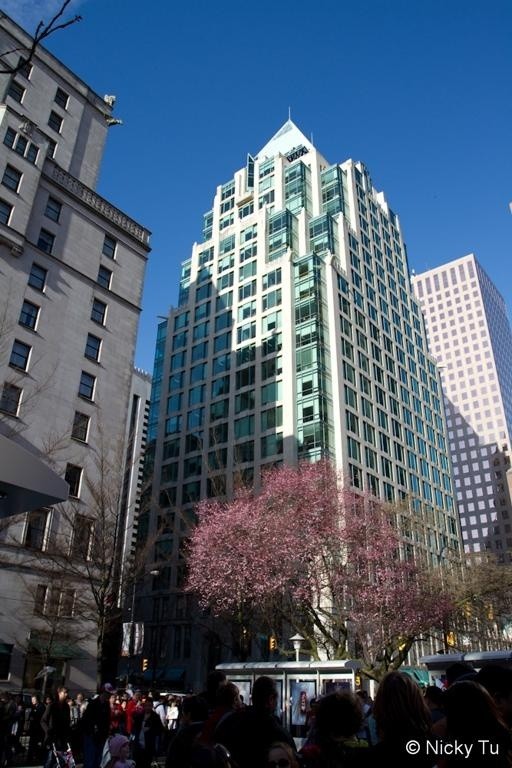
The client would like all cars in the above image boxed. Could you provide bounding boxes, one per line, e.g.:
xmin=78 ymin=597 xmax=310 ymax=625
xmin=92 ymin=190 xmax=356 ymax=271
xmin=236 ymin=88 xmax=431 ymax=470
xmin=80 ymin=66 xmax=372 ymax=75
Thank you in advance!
xmin=5 ymin=689 xmax=45 ymax=706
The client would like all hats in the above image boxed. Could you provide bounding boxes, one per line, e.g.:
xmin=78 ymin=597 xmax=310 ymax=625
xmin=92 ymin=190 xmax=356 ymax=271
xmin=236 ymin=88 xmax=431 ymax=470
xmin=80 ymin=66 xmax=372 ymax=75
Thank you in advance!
xmin=104 ymin=682 xmax=113 ymax=690
xmin=109 ymin=735 xmax=130 ymax=758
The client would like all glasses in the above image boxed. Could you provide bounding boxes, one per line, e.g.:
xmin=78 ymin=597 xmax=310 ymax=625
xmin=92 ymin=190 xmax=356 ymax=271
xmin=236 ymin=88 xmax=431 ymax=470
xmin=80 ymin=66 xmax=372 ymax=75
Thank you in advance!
xmin=269 ymin=758 xmax=291 ymax=768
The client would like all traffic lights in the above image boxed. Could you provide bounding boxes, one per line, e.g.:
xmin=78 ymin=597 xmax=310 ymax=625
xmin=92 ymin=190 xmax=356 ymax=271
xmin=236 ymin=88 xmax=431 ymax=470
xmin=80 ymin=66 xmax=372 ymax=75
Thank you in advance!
xmin=447 ymin=631 xmax=455 ymax=648
xmin=488 ymin=604 xmax=494 ymax=620
xmin=465 ymin=602 xmax=472 ymax=619
xmin=142 ymin=659 xmax=148 ymax=672
xmin=355 ymin=677 xmax=360 ymax=686
xmin=269 ymin=637 xmax=276 ymax=650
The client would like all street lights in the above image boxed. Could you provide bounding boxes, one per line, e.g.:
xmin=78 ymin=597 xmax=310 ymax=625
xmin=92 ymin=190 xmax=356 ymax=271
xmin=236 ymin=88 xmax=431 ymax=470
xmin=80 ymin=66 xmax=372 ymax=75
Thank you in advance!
xmin=289 ymin=632 xmax=306 ymax=683
xmin=115 ymin=568 xmax=161 ymax=692
